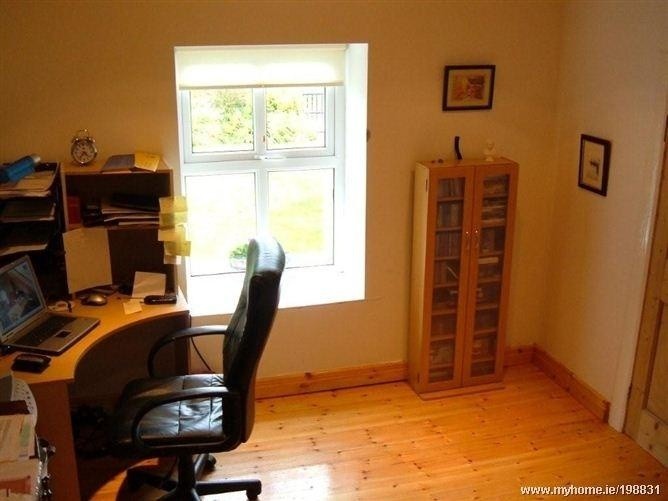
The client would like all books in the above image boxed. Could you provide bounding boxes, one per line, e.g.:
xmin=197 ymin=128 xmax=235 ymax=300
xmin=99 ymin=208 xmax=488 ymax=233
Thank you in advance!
xmin=0 ymin=414 xmax=45 ymax=501
xmin=428 ymin=174 xmax=498 ymax=384
xmin=98 ymin=197 xmax=166 ymax=226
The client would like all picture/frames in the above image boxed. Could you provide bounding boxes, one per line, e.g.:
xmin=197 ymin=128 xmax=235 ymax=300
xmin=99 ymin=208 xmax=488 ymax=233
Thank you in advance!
xmin=443 ymin=64 xmax=496 ymax=112
xmin=578 ymin=134 xmax=611 ymax=197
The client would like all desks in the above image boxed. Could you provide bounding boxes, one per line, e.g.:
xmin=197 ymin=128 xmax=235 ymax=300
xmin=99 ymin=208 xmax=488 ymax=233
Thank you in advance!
xmin=0 ymin=286 xmax=191 ymax=501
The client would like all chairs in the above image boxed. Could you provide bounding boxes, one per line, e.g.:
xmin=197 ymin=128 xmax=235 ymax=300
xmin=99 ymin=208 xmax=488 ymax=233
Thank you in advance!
xmin=113 ymin=234 xmax=285 ymax=501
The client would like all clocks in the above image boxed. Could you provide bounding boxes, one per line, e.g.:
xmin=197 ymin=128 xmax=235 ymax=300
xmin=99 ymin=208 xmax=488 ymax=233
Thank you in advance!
xmin=70 ymin=127 xmax=98 ymax=166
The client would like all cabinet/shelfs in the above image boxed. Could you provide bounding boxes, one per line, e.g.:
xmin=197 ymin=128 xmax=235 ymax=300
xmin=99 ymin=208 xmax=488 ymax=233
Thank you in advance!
xmin=61 ymin=156 xmax=180 ymax=295
xmin=406 ymin=156 xmax=520 ymax=397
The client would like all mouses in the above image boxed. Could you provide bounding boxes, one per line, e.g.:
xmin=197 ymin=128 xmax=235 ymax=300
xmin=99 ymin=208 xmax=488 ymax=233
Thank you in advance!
xmin=82 ymin=293 xmax=107 ymax=306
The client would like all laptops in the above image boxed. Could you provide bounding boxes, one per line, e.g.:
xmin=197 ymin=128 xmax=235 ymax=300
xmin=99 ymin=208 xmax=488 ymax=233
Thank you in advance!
xmin=0 ymin=256 xmax=100 ymax=356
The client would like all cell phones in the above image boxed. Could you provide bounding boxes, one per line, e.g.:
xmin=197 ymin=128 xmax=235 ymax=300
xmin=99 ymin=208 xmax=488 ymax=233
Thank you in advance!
xmin=144 ymin=295 xmax=176 ymax=304
